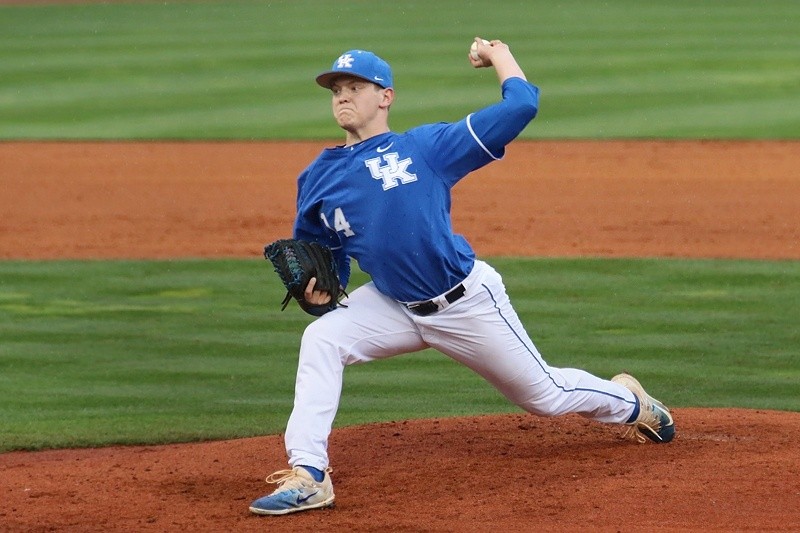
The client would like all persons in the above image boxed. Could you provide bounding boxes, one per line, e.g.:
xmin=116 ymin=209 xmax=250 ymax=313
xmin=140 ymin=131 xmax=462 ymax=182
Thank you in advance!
xmin=250 ymin=37 xmax=674 ymax=514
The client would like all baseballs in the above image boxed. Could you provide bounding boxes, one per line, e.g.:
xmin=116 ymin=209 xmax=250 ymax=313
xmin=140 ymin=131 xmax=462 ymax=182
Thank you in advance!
xmin=470 ymin=39 xmax=491 ymax=61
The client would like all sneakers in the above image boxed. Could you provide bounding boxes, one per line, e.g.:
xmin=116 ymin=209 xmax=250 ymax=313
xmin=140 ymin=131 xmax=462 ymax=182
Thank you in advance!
xmin=610 ymin=374 xmax=674 ymax=444
xmin=249 ymin=467 xmax=335 ymax=514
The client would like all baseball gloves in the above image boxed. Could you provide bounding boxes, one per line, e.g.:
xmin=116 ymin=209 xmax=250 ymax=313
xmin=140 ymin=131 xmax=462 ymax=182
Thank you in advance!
xmin=262 ymin=238 xmax=351 ymax=317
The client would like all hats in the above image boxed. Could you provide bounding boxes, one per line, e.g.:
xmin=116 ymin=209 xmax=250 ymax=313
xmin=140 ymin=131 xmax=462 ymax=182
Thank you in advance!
xmin=315 ymin=50 xmax=393 ymax=89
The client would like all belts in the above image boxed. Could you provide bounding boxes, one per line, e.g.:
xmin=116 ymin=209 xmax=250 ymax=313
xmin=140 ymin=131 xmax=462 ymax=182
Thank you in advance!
xmin=402 ymin=284 xmax=465 ymax=316
